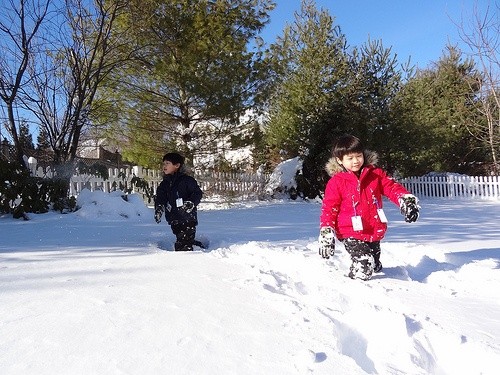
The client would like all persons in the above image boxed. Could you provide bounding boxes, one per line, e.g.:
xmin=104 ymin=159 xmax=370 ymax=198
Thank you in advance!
xmin=318 ymin=135 xmax=422 ymax=281
xmin=154 ymin=152 xmax=206 ymax=251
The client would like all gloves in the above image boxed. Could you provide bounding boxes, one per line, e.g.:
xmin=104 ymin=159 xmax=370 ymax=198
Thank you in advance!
xmin=177 ymin=201 xmax=194 ymax=216
xmin=399 ymin=197 xmax=420 ymax=223
xmin=318 ymin=228 xmax=336 ymax=259
xmin=154 ymin=206 xmax=164 ymax=224
